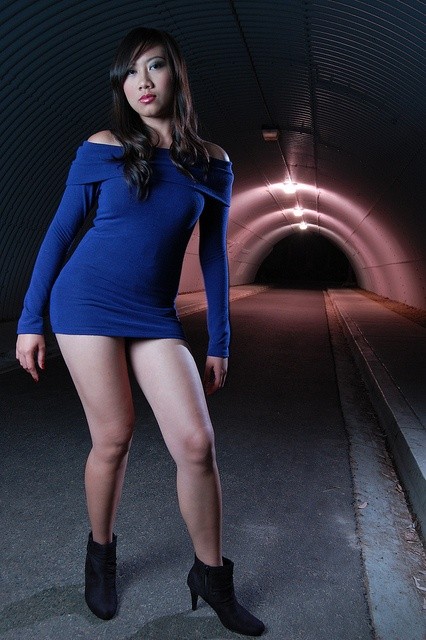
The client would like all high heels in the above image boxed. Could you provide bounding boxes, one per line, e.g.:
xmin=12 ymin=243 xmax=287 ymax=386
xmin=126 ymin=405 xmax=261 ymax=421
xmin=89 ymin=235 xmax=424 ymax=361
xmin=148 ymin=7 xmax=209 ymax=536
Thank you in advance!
xmin=187 ymin=553 xmax=265 ymax=636
xmin=85 ymin=531 xmax=118 ymax=619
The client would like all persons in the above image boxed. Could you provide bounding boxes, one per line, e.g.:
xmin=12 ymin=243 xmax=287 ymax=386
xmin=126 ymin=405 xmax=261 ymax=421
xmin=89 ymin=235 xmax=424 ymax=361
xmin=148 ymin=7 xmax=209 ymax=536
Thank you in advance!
xmin=15 ymin=26 xmax=265 ymax=636
